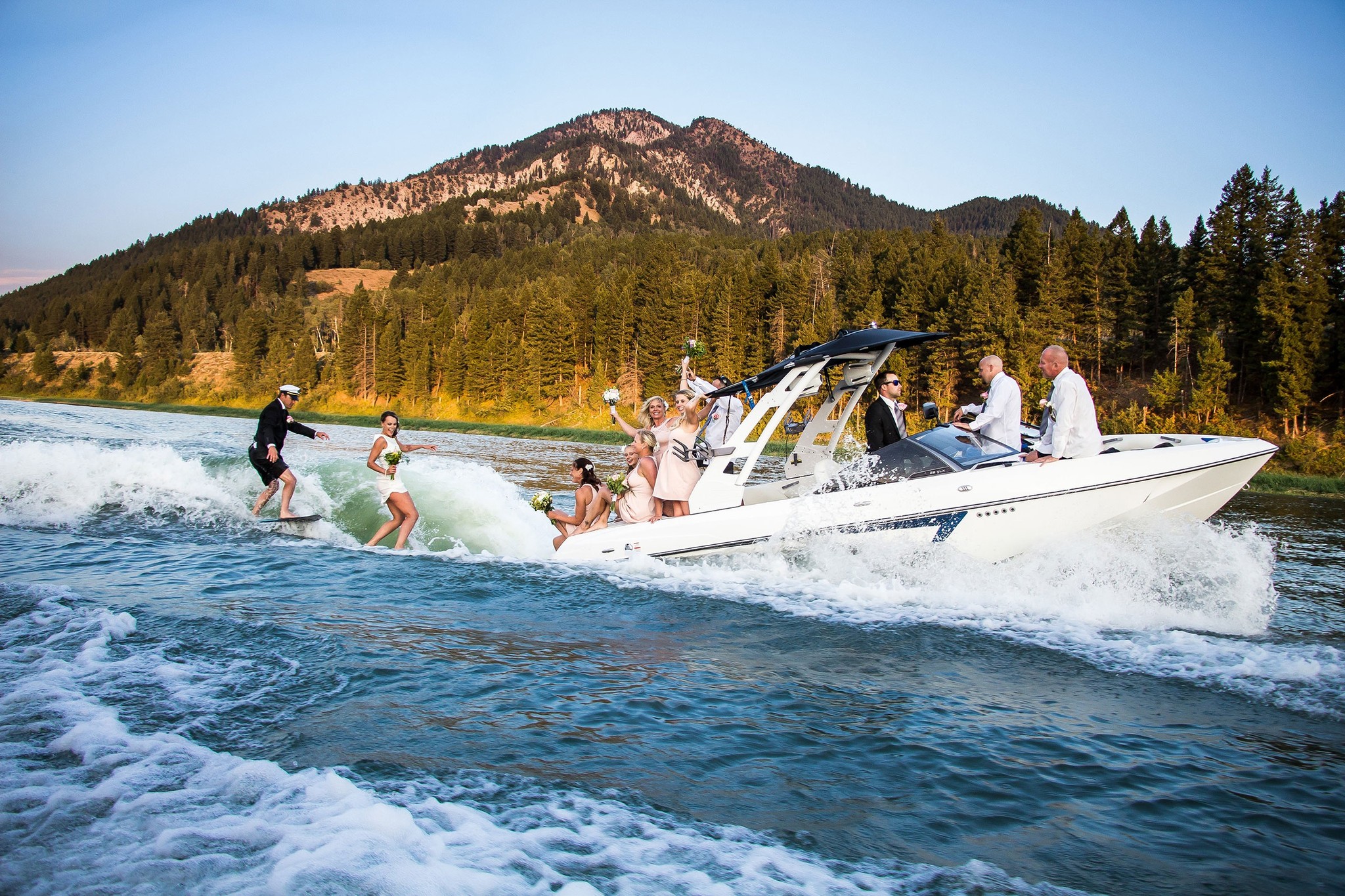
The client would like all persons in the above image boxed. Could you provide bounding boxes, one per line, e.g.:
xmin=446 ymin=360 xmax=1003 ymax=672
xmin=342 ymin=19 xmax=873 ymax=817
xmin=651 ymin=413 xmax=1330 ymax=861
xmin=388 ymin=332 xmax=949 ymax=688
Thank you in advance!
xmin=610 ymin=395 xmax=720 ymax=470
xmin=952 ymin=355 xmax=1021 ymax=456
xmin=249 ymin=384 xmax=330 ymax=519
xmin=614 ymin=444 xmax=639 ymax=520
xmin=674 ymin=364 xmax=743 ymax=464
xmin=367 ymin=411 xmax=438 ymax=550
xmin=570 ymin=430 xmax=663 ymax=536
xmin=1039 ymin=380 xmax=1055 ymax=441
xmin=546 ymin=458 xmax=607 ymax=552
xmin=652 ymin=357 xmax=713 ymax=517
xmin=1018 ymin=345 xmax=1103 ymax=467
xmin=865 ymin=370 xmax=923 ymax=475
xmin=955 ymin=435 xmax=982 ymax=448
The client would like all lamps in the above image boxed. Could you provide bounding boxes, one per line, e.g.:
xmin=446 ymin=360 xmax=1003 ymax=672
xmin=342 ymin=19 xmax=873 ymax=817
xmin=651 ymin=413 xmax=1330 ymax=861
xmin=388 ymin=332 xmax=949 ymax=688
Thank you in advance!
xmin=843 ymin=363 xmax=874 ymax=385
xmin=789 ymin=373 xmax=823 ymax=395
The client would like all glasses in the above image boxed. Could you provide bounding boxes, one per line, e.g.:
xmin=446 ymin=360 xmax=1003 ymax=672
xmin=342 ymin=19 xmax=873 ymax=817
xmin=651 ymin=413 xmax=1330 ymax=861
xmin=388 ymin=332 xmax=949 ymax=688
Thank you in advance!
xmin=1048 ymin=406 xmax=1057 ymax=422
xmin=879 ymin=380 xmax=900 ymax=389
xmin=721 ymin=376 xmax=728 ymax=386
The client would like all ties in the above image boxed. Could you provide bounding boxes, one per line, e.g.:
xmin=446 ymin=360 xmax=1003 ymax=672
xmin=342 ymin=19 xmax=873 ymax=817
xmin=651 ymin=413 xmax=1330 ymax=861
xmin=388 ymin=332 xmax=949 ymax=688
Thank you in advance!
xmin=894 ymin=403 xmax=907 ymax=439
xmin=977 ymin=386 xmax=992 ymax=434
xmin=695 ymin=405 xmax=719 ymax=442
xmin=1039 ymin=384 xmax=1055 ymax=438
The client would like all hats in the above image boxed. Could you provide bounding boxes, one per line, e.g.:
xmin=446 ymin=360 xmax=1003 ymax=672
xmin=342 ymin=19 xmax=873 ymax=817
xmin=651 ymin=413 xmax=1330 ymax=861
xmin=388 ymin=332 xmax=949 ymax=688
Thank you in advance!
xmin=280 ymin=385 xmax=302 ymax=402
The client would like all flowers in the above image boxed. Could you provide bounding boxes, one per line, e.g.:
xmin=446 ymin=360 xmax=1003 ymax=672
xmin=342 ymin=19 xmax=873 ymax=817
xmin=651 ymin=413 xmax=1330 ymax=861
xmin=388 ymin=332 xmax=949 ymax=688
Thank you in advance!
xmin=602 ymin=385 xmax=621 ymax=424
xmin=607 ymin=471 xmax=630 ymax=512
xmin=664 ymin=401 xmax=669 ymax=411
xmin=676 ymin=336 xmax=706 ymax=373
xmin=287 ymin=416 xmax=294 ymax=423
xmin=980 ymin=391 xmax=989 ymax=403
xmin=530 ymin=490 xmax=558 ymax=525
xmin=1039 ymin=398 xmax=1054 ymax=414
xmin=622 ymin=444 xmax=627 ymax=451
xmin=585 ymin=464 xmax=593 ymax=471
xmin=897 ymin=402 xmax=907 ymax=415
xmin=653 ymin=441 xmax=660 ymax=454
xmin=712 ymin=414 xmax=719 ymax=423
xmin=384 ymin=452 xmax=402 ymax=480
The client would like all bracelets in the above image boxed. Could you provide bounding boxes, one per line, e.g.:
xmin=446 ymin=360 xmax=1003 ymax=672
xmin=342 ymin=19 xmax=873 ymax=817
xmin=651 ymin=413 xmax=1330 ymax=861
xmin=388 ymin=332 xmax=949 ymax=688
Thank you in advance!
xmin=681 ymin=379 xmax=687 ymax=380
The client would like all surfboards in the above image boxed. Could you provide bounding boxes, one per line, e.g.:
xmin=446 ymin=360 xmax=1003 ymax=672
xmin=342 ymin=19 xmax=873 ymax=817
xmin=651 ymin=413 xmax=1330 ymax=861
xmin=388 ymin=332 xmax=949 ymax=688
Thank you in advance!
xmin=258 ymin=514 xmax=323 ymax=522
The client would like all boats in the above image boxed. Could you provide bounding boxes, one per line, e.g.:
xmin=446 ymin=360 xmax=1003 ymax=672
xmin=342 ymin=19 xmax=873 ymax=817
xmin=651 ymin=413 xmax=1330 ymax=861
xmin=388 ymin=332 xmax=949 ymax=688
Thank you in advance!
xmin=546 ymin=323 xmax=1279 ymax=575
